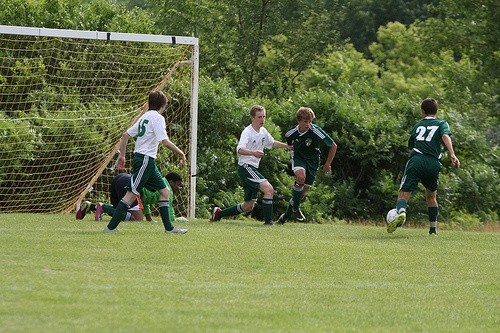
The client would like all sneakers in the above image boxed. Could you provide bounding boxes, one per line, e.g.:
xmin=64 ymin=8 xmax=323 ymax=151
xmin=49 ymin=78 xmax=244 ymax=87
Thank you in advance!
xmin=387 ymin=212 xmax=406 ymax=233
xmin=292 ymin=208 xmax=306 ymax=222
xmin=275 ymin=213 xmax=287 ymax=225
xmin=264 ymin=221 xmax=277 ymax=225
xmin=429 ymin=229 xmax=438 ymax=237
xmin=76 ymin=200 xmax=91 ymax=220
xmin=103 ymin=227 xmax=120 ymax=232
xmin=165 ymin=226 xmax=188 ymax=234
xmin=95 ymin=202 xmax=105 ymax=221
xmin=210 ymin=207 xmax=222 ymax=221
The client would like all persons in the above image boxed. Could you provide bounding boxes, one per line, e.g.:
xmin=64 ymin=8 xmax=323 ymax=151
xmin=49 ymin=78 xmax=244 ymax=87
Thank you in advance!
xmin=212 ymin=105 xmax=293 ymax=226
xmin=76 ymin=172 xmax=188 ymax=223
xmin=387 ymin=99 xmax=460 ymax=236
xmin=275 ymin=107 xmax=337 ymax=225
xmin=102 ymin=91 xmax=188 ymax=234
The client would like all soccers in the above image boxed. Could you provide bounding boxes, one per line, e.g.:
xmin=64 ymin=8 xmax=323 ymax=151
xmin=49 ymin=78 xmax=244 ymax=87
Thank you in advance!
xmin=386 ymin=208 xmax=406 ymax=226
